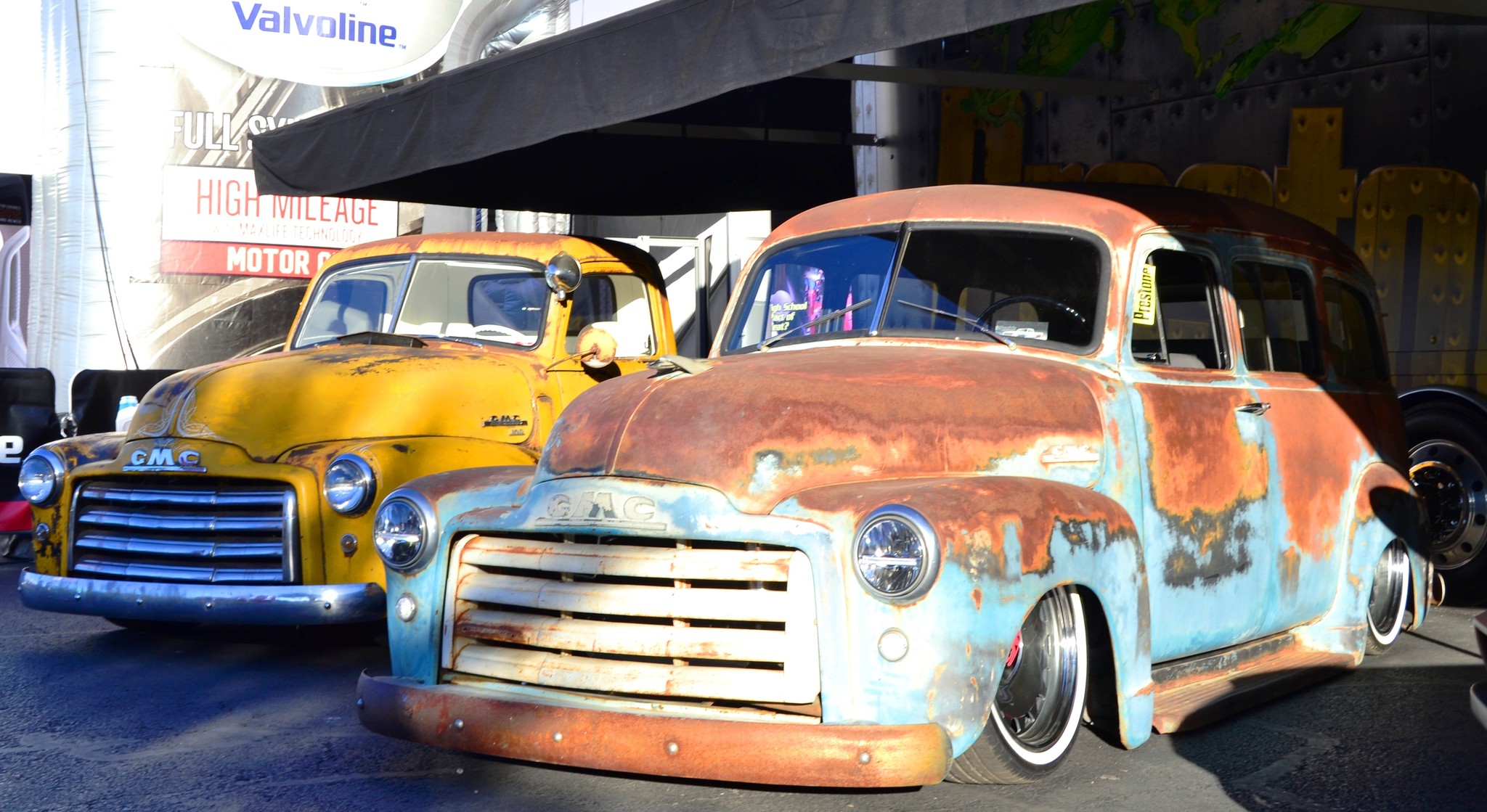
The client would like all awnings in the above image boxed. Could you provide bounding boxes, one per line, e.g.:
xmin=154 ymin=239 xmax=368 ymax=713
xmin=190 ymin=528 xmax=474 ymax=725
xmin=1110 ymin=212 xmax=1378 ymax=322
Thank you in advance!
xmin=255 ymin=2 xmax=1487 ymax=217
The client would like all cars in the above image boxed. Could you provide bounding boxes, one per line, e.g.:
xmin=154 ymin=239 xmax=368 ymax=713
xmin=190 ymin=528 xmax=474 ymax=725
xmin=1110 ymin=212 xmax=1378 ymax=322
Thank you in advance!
xmin=354 ymin=177 xmax=1440 ymax=796
xmin=17 ymin=229 xmax=677 ymax=623
